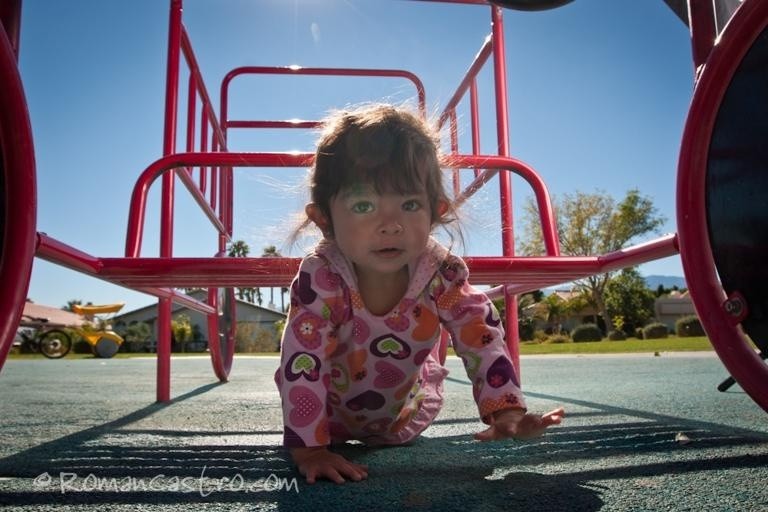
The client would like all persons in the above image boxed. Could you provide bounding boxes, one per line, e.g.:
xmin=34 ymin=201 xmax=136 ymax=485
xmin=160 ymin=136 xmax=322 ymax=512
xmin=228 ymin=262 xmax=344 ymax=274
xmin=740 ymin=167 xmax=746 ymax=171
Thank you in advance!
xmin=272 ymin=98 xmax=565 ymax=484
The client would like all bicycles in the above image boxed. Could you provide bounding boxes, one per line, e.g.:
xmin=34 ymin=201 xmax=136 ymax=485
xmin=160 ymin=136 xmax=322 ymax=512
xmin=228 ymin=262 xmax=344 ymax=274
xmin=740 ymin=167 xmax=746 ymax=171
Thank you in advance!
xmin=7 ymin=312 xmax=73 ymax=363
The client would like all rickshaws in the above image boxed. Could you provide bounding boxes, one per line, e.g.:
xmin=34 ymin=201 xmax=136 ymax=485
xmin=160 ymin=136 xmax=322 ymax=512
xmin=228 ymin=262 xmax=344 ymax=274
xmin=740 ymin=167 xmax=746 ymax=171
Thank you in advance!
xmin=72 ymin=300 xmax=127 ymax=357
